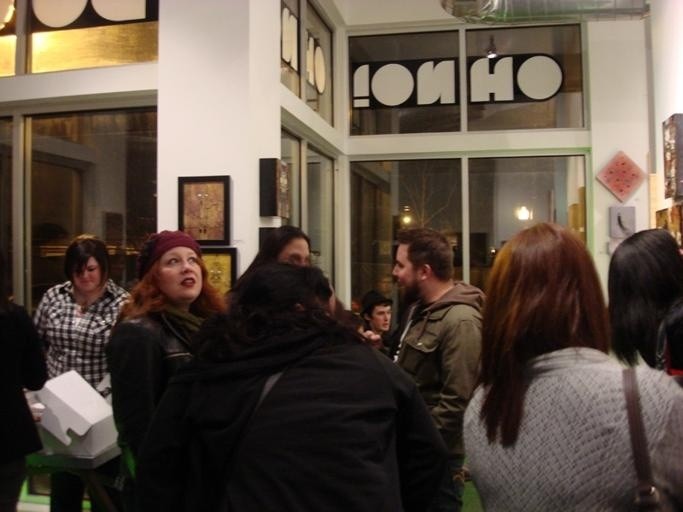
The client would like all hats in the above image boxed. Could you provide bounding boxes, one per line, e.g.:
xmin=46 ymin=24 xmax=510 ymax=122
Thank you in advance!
xmin=360 ymin=291 xmax=394 ymax=317
xmin=137 ymin=231 xmax=202 ymax=282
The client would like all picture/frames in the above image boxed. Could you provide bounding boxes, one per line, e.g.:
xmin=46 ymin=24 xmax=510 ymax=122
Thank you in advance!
xmin=178 ymin=176 xmax=238 ymax=294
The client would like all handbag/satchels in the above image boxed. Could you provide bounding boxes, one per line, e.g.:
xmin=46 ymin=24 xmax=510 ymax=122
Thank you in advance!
xmin=631 ymin=487 xmax=682 ymax=512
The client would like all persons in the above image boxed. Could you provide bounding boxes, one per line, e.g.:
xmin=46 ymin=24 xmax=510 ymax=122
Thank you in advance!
xmin=393 ymin=228 xmax=486 ymax=511
xmin=33 ymin=236 xmax=132 ymax=511
xmin=1 ymin=254 xmax=48 ymax=512
xmin=111 ymin=222 xmax=448 ymax=511
xmin=464 ymin=222 xmax=682 ymax=511
